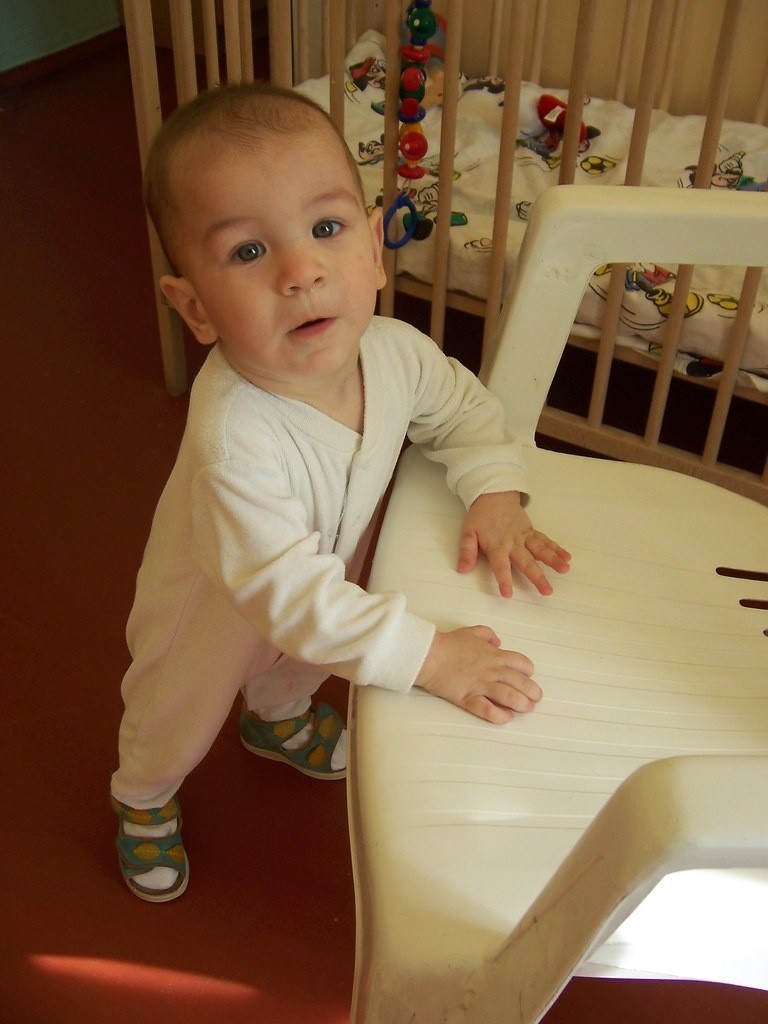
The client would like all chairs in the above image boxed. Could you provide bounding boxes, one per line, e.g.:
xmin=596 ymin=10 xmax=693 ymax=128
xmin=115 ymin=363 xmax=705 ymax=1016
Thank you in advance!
xmin=313 ymin=182 xmax=767 ymax=1024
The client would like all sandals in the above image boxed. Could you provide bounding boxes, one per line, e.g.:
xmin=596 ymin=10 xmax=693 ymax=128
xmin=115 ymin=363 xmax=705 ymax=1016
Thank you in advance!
xmin=111 ymin=796 xmax=187 ymax=903
xmin=239 ymin=703 xmax=350 ymax=780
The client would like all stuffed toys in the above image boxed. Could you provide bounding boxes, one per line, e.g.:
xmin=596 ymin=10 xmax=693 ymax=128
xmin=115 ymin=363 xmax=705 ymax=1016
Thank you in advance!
xmin=519 ymin=93 xmax=587 ymax=150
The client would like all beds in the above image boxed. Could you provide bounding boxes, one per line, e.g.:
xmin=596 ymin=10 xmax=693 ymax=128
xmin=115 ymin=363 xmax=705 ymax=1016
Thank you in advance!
xmin=111 ymin=1 xmax=768 ymax=504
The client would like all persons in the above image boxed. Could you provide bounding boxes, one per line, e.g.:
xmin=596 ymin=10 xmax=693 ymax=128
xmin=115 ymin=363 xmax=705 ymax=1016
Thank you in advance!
xmin=110 ymin=82 xmax=571 ymax=903
